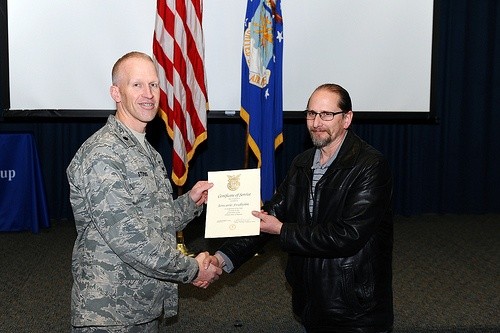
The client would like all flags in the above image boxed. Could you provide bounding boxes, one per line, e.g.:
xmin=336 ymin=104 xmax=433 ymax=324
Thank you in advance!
xmin=153 ymin=0 xmax=209 ymax=187
xmin=241 ymin=0 xmax=285 ymax=204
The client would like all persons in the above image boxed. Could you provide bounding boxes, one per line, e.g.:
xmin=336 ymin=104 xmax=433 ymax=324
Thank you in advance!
xmin=66 ymin=49 xmax=226 ymax=333
xmin=195 ymin=82 xmax=396 ymax=333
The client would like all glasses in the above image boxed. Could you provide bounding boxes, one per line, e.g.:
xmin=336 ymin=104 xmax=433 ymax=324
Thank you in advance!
xmin=303 ymin=109 xmax=345 ymax=120
